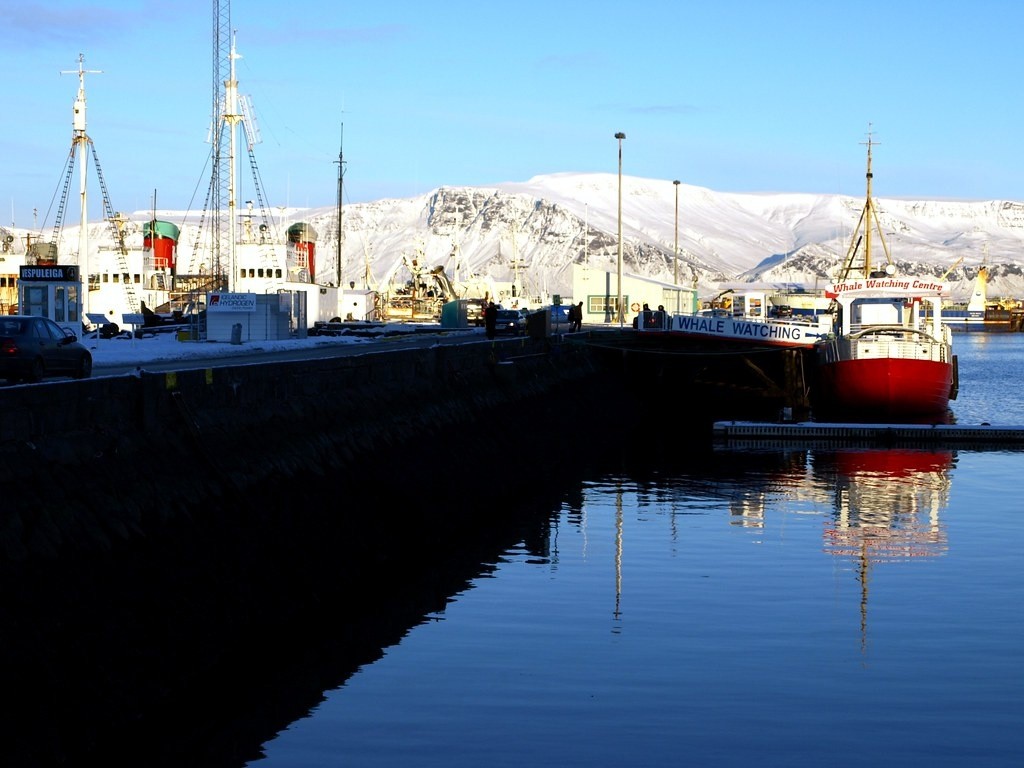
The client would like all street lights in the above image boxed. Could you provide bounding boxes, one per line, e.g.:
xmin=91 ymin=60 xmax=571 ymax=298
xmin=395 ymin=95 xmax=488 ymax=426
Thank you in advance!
xmin=613 ymin=132 xmax=626 ymax=328
xmin=673 ymin=179 xmax=681 ymax=284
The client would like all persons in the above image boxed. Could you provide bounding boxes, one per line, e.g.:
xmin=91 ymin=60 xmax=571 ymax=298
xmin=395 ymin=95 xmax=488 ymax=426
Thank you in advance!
xmin=828 ymin=297 xmax=845 ymax=338
xmin=485 ymin=301 xmax=498 ymax=339
xmin=567 ymin=301 xmax=583 ymax=334
xmin=643 ymin=302 xmax=668 ymax=328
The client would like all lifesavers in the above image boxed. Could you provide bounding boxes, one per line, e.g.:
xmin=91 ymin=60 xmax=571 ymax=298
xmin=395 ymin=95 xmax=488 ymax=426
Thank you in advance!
xmin=631 ymin=302 xmax=641 ymax=312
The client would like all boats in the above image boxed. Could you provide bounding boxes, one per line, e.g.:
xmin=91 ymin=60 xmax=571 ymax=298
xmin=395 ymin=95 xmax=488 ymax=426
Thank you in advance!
xmin=768 ymin=294 xmax=834 ymax=318
xmin=813 ymin=123 xmax=961 ymax=420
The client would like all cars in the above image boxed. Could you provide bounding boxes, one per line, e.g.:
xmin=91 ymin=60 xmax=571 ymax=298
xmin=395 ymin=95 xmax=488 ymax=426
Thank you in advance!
xmin=1 ymin=315 xmax=93 ymax=384
xmin=496 ymin=309 xmax=527 ymax=337
